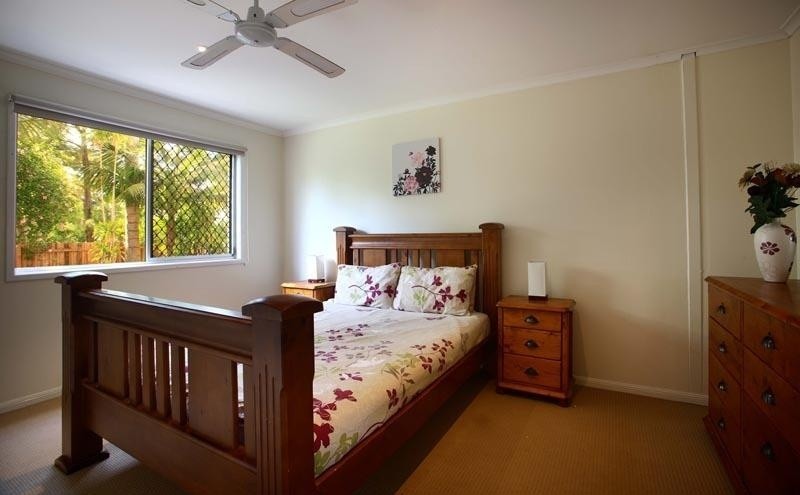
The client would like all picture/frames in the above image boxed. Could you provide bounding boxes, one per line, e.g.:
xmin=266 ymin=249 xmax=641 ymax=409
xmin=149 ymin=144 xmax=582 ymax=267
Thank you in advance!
xmin=391 ymin=138 xmax=440 ymax=196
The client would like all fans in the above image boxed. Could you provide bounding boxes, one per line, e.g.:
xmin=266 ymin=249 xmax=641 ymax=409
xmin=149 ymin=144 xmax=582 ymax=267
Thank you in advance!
xmin=180 ymin=0 xmax=353 ymax=77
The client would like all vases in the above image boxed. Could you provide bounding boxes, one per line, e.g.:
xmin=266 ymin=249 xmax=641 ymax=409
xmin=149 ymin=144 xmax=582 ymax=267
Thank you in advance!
xmin=753 ymin=217 xmax=797 ymax=282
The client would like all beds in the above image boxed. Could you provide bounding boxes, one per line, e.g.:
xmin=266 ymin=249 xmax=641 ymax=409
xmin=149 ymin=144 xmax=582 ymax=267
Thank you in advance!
xmin=53 ymin=223 xmax=501 ymax=493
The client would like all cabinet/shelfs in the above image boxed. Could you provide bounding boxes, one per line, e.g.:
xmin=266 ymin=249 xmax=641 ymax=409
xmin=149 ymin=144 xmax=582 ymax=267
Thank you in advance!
xmin=705 ymin=277 xmax=797 ymax=495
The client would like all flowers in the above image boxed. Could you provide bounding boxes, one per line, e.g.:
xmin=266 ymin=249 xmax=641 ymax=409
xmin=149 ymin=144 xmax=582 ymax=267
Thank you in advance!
xmin=739 ymin=162 xmax=799 ymax=234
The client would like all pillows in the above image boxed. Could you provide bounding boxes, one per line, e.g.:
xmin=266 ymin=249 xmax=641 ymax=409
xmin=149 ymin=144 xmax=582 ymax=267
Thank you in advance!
xmin=395 ymin=265 xmax=478 ymax=316
xmin=335 ymin=262 xmax=398 ymax=311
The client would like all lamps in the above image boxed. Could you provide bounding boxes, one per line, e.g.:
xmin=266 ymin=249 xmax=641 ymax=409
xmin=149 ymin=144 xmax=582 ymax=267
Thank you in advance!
xmin=527 ymin=261 xmax=547 ymax=300
xmin=308 ymin=255 xmax=325 ymax=282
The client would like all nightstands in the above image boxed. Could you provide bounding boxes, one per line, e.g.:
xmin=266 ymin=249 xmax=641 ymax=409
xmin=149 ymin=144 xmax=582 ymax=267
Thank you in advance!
xmin=282 ymin=283 xmax=334 ymax=300
xmin=499 ymin=295 xmax=575 ymax=408
